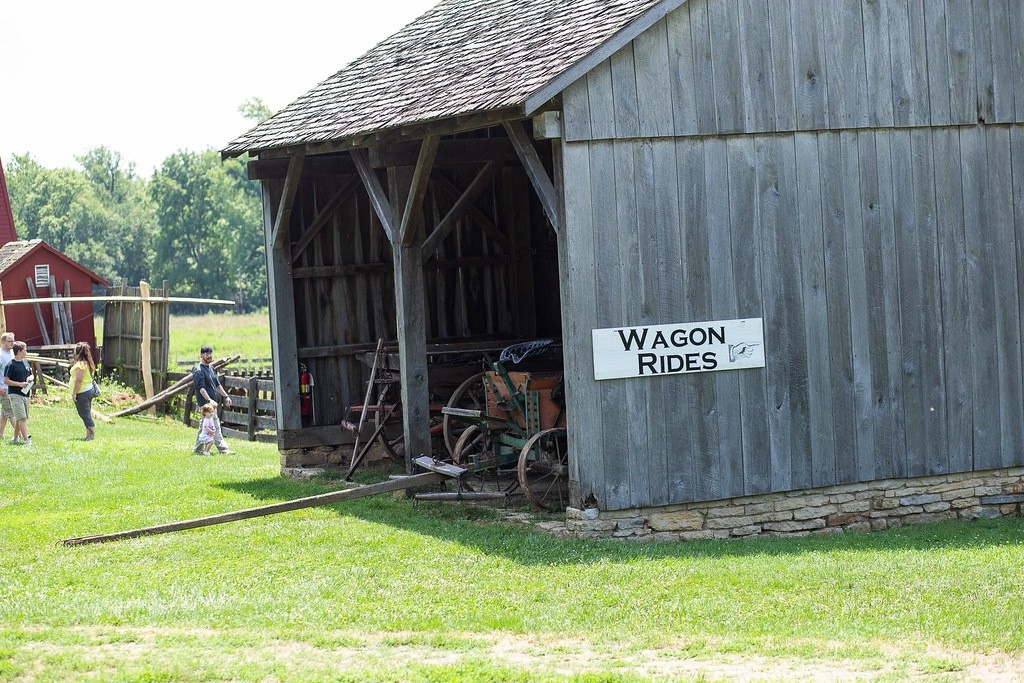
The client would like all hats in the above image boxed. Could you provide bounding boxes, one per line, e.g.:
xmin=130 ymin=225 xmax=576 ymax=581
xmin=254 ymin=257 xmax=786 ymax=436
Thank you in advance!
xmin=201 ymin=345 xmax=213 ymax=353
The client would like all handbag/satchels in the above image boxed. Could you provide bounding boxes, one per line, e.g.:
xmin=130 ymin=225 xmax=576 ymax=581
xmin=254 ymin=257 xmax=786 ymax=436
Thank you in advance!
xmin=92 ymin=380 xmax=102 ymax=397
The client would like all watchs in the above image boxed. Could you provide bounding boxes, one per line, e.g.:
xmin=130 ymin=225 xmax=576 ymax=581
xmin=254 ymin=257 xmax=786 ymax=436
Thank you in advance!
xmin=224 ymin=396 xmax=229 ymax=399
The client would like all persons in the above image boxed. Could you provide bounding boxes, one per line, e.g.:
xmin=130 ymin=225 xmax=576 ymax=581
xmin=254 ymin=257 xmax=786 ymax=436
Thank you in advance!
xmin=192 ymin=345 xmax=236 ymax=457
xmin=69 ymin=341 xmax=100 ymax=442
xmin=0 ymin=333 xmax=32 ymax=440
xmin=200 ymin=405 xmax=216 ymax=455
xmin=4 ymin=341 xmax=35 ymax=444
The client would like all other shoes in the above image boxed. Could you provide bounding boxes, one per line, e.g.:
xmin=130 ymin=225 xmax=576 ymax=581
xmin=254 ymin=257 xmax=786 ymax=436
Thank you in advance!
xmin=0 ymin=432 xmax=33 ymax=446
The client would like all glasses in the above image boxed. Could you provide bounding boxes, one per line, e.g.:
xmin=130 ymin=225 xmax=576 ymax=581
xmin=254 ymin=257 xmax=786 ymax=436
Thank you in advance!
xmin=201 ymin=351 xmax=212 ymax=354
xmin=5 ymin=339 xmax=16 ymax=343
